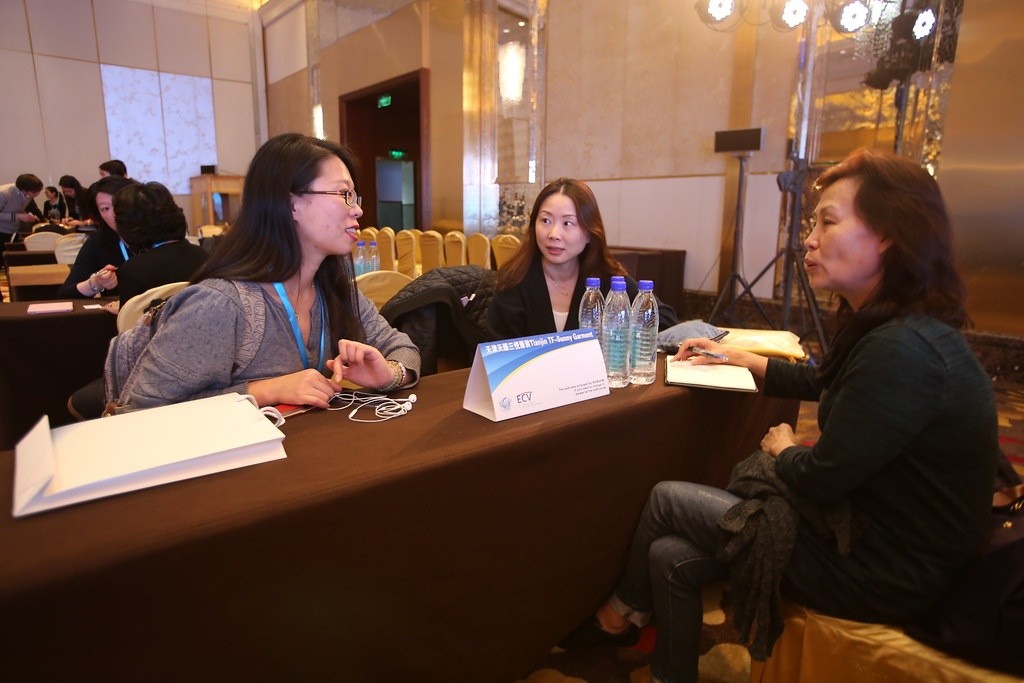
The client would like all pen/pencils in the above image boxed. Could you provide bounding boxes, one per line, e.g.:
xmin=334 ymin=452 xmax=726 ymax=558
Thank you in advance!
xmin=676 ymin=342 xmax=728 ymax=362
xmin=92 ymin=267 xmax=118 ymax=277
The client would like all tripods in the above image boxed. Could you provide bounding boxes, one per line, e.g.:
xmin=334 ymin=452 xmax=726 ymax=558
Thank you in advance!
xmin=707 ymin=138 xmax=831 ymax=361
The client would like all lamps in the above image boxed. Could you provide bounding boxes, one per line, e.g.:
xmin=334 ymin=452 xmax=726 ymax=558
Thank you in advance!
xmin=892 ymin=8 xmax=936 ymax=40
xmin=695 ymin=0 xmax=733 ymax=23
xmin=828 ymin=0 xmax=869 ymax=33
xmin=769 ymin=0 xmax=807 ymax=29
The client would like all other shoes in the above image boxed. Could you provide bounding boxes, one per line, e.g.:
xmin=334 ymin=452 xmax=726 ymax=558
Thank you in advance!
xmin=556 ymin=612 xmax=641 ymax=651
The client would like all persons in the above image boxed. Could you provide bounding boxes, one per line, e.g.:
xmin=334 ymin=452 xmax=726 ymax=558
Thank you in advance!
xmin=480 ymin=180 xmax=676 ymax=334
xmin=550 ymin=149 xmax=999 ymax=683
xmin=0 ymin=173 xmax=95 ymax=242
xmin=59 ymin=175 xmax=134 ymax=299
xmin=97 ymin=161 xmax=127 ymax=178
xmin=104 ymin=182 xmax=204 ymax=317
xmin=105 ymin=133 xmax=421 ymax=428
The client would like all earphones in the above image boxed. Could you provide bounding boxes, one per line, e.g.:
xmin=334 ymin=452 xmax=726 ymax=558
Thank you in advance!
xmin=394 ymin=394 xmax=417 ymax=403
xmin=391 ymin=401 xmax=412 ymax=411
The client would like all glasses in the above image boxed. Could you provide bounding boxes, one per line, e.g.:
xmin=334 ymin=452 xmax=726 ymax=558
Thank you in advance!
xmin=292 ymin=190 xmax=362 ymax=208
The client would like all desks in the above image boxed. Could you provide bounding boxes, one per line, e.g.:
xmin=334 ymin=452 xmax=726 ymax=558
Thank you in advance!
xmin=0 ymin=297 xmax=124 ymax=450
xmin=3 ymin=251 xmax=58 ymax=267
xmin=607 ymin=245 xmax=687 ymax=316
xmin=6 ymin=263 xmax=75 ymax=303
xmin=0 ymin=346 xmax=802 ymax=683
xmin=4 ymin=242 xmax=26 ymax=252
xmin=189 ymin=176 xmax=248 ymax=237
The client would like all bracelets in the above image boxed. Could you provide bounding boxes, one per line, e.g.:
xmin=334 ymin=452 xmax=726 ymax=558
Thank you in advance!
xmin=377 ymin=361 xmax=407 ymax=391
xmin=89 ymin=273 xmax=105 ymax=292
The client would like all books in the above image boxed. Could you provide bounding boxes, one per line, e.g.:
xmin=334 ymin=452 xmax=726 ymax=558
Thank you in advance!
xmin=665 ymin=353 xmax=758 ymax=393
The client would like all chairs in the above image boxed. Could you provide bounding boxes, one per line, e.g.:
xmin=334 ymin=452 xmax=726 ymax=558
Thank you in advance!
xmin=351 ymin=227 xmax=521 ymax=314
xmin=55 ymin=234 xmax=90 ymax=265
xmin=116 ymin=281 xmax=189 ymax=336
xmin=22 ymin=232 xmax=63 ymax=252
xmin=746 ymin=598 xmax=1024 ymax=683
xmin=197 ymin=224 xmax=223 ymax=238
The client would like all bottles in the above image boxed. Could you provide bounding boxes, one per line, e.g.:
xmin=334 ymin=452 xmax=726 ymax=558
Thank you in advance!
xmin=602 ymin=282 xmax=632 ymax=388
xmin=353 ymin=242 xmax=367 ymax=278
xmin=630 ymin=281 xmax=659 ymax=385
xmin=578 ymin=278 xmax=606 ymax=349
xmin=606 ymin=276 xmax=630 ymax=306
xmin=365 ymin=242 xmax=381 ymax=274
